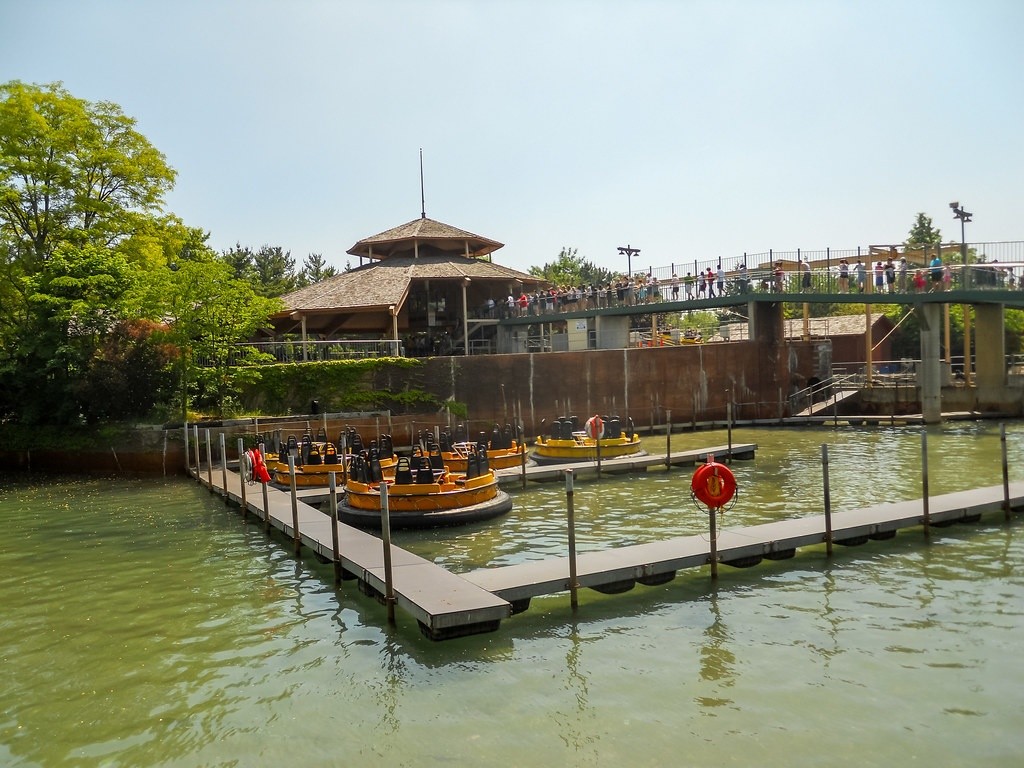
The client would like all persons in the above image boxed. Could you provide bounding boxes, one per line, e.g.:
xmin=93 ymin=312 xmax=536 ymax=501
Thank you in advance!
xmin=387 ymin=255 xmax=1024 ymax=356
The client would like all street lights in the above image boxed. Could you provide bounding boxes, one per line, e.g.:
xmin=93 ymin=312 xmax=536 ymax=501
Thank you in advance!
xmin=617 ymin=244 xmax=640 ymax=278
xmin=949 ymin=202 xmax=972 ymax=291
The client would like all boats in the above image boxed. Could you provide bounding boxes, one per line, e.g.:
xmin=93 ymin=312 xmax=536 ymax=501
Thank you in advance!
xmin=255 ymin=427 xmax=399 ymax=491
xmin=529 ymin=415 xmax=648 ymax=466
xmin=337 ymin=444 xmax=512 ymax=529
xmin=416 ymin=423 xmax=530 ymax=472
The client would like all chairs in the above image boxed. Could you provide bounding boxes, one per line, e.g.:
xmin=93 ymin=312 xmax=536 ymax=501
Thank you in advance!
xmin=255 ymin=427 xmax=490 ymax=484
xmin=418 ymin=423 xmax=523 ymax=452
xmin=541 ymin=415 xmax=634 ymax=445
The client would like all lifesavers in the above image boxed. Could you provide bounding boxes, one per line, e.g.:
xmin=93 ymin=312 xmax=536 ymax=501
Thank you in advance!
xmin=584 ymin=417 xmax=606 ymax=441
xmin=691 ymin=461 xmax=737 ymax=507
xmin=243 ymin=449 xmax=272 ymax=486
